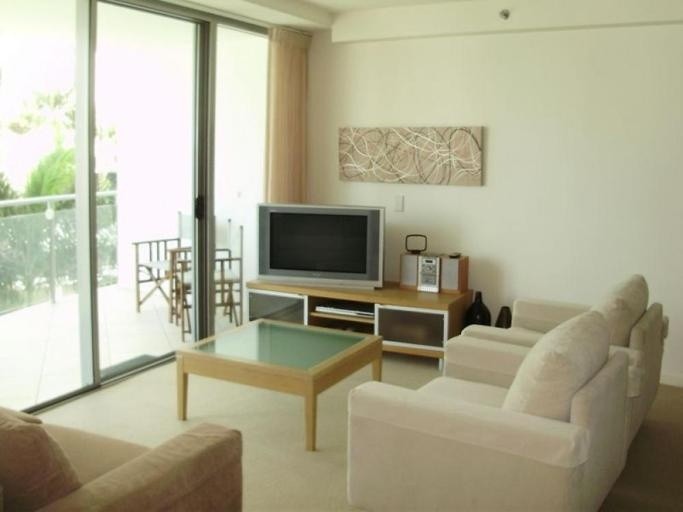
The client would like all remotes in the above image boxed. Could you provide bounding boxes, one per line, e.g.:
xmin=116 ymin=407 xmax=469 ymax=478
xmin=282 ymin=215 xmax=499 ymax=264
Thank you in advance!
xmin=449 ymin=252 xmax=460 ymax=258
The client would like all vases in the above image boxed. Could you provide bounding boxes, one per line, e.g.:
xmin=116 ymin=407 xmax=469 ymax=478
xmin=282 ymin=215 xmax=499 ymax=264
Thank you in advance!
xmin=495 ymin=307 xmax=511 ymax=328
xmin=465 ymin=291 xmax=490 ymax=327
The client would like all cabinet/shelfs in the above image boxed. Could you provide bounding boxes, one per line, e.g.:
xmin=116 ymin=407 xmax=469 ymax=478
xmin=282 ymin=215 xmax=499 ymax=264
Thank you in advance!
xmin=246 ymin=278 xmax=473 ymax=371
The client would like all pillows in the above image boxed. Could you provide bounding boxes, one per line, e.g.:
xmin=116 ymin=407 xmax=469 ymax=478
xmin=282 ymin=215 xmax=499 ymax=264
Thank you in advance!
xmin=0 ymin=407 xmax=83 ymax=512
xmin=590 ymin=274 xmax=648 ymax=347
xmin=503 ymin=311 xmax=610 ymax=422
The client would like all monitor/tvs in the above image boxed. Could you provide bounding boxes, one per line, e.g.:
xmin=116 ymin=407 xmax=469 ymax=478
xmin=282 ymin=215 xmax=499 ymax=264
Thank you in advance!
xmin=258 ymin=204 xmax=384 ymax=291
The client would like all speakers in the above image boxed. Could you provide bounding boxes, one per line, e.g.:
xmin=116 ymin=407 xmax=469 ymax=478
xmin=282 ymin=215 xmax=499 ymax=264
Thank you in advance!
xmin=439 ymin=255 xmax=469 ymax=294
xmin=399 ymin=253 xmax=418 ymax=289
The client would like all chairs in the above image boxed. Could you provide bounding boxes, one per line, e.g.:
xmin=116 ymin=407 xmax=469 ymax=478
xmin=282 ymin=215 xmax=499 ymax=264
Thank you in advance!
xmin=133 ymin=238 xmax=181 ymax=312
xmin=177 ymin=258 xmax=243 ymax=342
xmin=0 ymin=407 xmax=242 ymax=512
xmin=348 ymin=335 xmax=629 ymax=512
xmin=461 ymin=296 xmax=669 ymax=450
